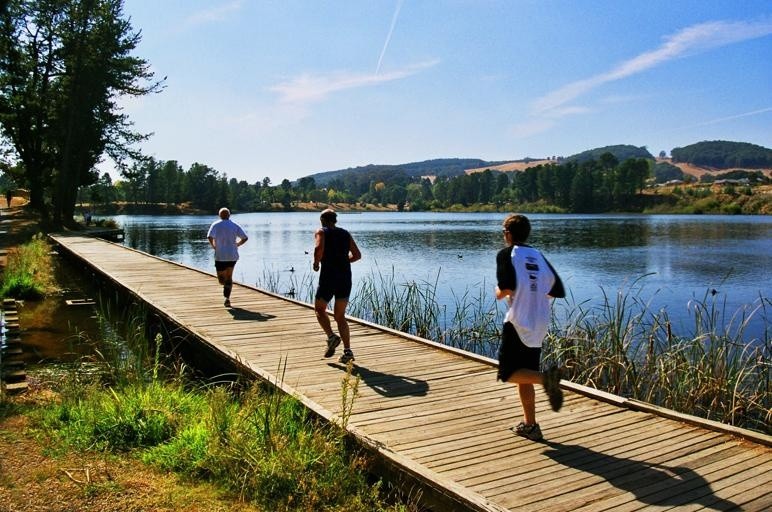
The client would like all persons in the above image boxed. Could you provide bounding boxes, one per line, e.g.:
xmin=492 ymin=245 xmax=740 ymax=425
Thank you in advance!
xmin=206 ymin=206 xmax=249 ymax=307
xmin=312 ymin=208 xmax=361 ymax=363
xmin=494 ymin=214 xmax=567 ymax=443
xmin=6 ymin=188 xmax=12 ymax=208
xmin=83 ymin=210 xmax=90 ymax=223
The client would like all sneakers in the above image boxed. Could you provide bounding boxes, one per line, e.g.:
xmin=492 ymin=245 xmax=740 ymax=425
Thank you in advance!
xmin=514 ymin=422 xmax=543 ymax=440
xmin=324 ymin=335 xmax=341 ymax=358
xmin=338 ymin=350 xmax=355 ymax=363
xmin=544 ymin=366 xmax=563 ymax=412
